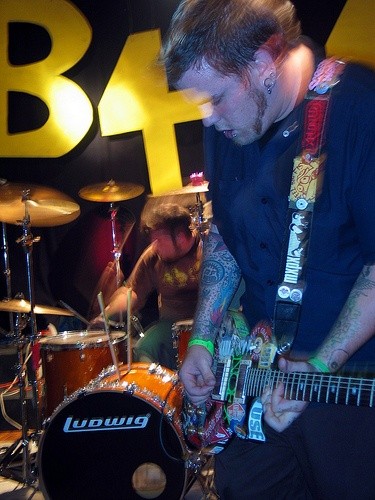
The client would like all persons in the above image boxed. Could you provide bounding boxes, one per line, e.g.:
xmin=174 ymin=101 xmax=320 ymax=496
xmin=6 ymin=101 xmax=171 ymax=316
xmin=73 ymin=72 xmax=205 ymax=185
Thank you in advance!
xmin=90 ymin=203 xmax=203 ymax=372
xmin=151 ymin=0 xmax=375 ymax=500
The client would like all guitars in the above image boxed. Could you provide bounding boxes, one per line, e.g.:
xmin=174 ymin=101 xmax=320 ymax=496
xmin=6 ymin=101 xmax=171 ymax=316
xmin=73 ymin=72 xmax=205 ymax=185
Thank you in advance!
xmin=175 ymin=311 xmax=374 ymax=455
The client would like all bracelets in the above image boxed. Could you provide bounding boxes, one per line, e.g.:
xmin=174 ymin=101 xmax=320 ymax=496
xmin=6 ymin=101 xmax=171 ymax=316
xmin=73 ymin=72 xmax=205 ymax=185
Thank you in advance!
xmin=304 ymin=355 xmax=332 ymax=375
xmin=186 ymin=338 xmax=216 ymax=360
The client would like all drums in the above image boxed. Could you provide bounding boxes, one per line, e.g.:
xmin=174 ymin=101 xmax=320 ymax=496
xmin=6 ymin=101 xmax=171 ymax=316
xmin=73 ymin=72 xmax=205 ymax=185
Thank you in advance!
xmin=38 ymin=331 xmax=129 ymax=411
xmin=36 ymin=361 xmax=210 ymax=499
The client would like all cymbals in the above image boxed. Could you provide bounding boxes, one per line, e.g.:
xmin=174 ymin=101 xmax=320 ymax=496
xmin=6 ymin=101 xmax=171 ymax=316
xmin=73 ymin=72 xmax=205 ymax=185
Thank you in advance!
xmin=0 ymin=299 xmax=72 ymax=318
xmin=0 ymin=186 xmax=80 ymax=226
xmin=147 ymin=178 xmax=209 ymax=198
xmin=79 ymin=182 xmax=144 ymax=202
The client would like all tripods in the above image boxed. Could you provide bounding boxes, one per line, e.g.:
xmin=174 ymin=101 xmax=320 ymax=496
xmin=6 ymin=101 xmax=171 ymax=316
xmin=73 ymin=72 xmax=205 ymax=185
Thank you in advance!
xmin=0 ymin=197 xmax=47 ymax=483
